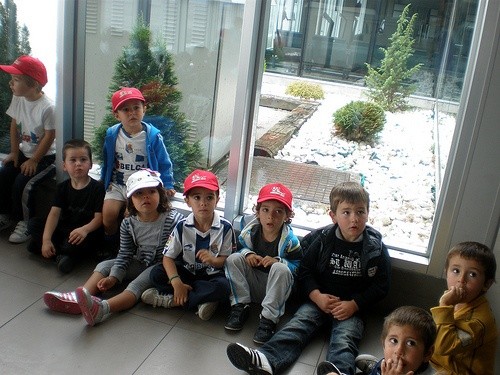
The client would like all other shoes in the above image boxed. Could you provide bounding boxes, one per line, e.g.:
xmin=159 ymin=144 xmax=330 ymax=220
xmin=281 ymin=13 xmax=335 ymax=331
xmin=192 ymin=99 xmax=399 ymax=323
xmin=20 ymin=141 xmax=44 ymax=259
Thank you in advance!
xmin=36 ymin=251 xmax=72 ymax=273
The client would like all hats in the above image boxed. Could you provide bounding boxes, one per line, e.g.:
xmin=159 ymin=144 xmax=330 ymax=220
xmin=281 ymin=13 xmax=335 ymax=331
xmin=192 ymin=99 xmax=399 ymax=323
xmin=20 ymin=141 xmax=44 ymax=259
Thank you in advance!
xmin=183 ymin=170 xmax=218 ymax=196
xmin=112 ymin=87 xmax=146 ymax=110
xmin=0 ymin=55 xmax=48 ymax=87
xmin=257 ymin=183 xmax=293 ymax=211
xmin=126 ymin=168 xmax=163 ymax=199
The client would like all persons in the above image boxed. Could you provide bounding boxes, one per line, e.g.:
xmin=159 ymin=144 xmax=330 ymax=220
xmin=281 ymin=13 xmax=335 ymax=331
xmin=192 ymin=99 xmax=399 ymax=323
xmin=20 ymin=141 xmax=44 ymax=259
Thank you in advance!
xmin=317 ymin=306 xmax=436 ymax=375
xmin=224 ymin=182 xmax=302 ymax=344
xmin=43 ymin=168 xmax=185 ymax=327
xmin=26 ymin=139 xmax=105 ymax=274
xmin=99 ymin=87 xmax=176 ymax=263
xmin=141 ymin=168 xmax=236 ymax=321
xmin=429 ymin=241 xmax=498 ymax=375
xmin=0 ymin=55 xmax=56 ymax=245
xmin=226 ymin=181 xmax=391 ymax=375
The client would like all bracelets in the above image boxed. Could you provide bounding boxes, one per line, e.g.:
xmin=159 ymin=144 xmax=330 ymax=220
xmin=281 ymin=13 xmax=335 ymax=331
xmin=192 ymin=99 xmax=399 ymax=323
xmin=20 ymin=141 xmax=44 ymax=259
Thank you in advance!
xmin=169 ymin=275 xmax=179 ymax=284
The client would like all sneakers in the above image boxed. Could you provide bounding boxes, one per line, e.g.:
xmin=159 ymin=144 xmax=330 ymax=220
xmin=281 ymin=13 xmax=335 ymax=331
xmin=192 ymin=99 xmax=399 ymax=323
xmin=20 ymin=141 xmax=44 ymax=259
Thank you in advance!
xmin=226 ymin=343 xmax=273 ymax=375
xmin=0 ymin=215 xmax=10 ymax=231
xmin=317 ymin=361 xmax=342 ymax=375
xmin=43 ymin=291 xmax=84 ymax=314
xmin=355 ymin=353 xmax=380 ymax=374
xmin=198 ymin=302 xmax=219 ymax=320
xmin=76 ymin=287 xmax=104 ymax=328
xmin=224 ymin=303 xmax=251 ymax=331
xmin=141 ymin=288 xmax=174 ymax=309
xmin=252 ymin=314 xmax=277 ymax=344
xmin=8 ymin=221 xmax=32 ymax=243
